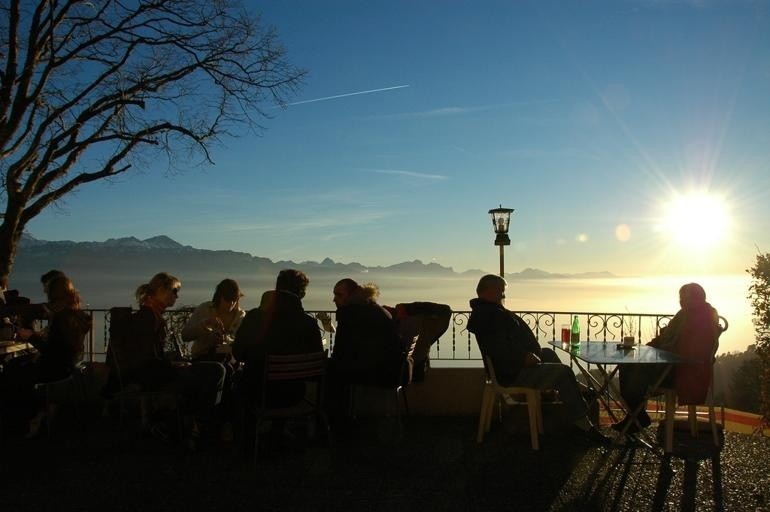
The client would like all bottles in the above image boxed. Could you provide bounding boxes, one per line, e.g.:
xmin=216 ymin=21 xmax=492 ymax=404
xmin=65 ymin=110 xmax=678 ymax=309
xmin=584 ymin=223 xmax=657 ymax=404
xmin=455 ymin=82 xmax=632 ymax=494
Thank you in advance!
xmin=570 ymin=315 xmax=580 ymax=348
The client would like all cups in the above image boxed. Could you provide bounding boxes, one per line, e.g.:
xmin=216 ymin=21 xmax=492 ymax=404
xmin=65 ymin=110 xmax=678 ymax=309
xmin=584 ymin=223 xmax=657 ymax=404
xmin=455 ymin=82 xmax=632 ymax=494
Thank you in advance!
xmin=562 ymin=324 xmax=571 ymax=345
xmin=623 ymin=337 xmax=634 ymax=345
xmin=624 ymin=349 xmax=635 ymax=359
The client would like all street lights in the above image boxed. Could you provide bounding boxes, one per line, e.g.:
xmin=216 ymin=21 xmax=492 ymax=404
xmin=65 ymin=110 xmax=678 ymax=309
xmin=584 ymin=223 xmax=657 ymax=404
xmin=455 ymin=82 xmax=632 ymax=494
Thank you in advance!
xmin=487 ymin=204 xmax=516 ymax=276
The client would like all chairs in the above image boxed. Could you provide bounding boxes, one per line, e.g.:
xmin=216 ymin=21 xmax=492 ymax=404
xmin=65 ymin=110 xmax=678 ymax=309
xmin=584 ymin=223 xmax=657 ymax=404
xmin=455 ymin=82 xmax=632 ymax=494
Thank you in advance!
xmin=0 ymin=304 xmax=422 ymax=484
xmin=475 ymin=349 xmax=544 ymax=452
xmin=643 ymin=326 xmax=720 ymax=456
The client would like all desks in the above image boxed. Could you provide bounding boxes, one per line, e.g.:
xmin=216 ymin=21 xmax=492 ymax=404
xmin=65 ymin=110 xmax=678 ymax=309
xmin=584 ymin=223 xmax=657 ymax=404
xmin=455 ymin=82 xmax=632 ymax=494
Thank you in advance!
xmin=548 ymin=339 xmax=686 ymax=464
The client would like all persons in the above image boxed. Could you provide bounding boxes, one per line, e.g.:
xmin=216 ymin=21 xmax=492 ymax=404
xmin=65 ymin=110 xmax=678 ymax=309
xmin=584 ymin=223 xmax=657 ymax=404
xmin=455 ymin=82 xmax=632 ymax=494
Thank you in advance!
xmin=182 ymin=280 xmax=244 ymax=453
xmin=232 ymin=269 xmax=322 ymax=474
xmin=467 ymin=275 xmax=612 ymax=444
xmin=611 ymin=284 xmax=716 ymax=434
xmin=381 ymin=302 xmax=453 ymax=385
xmin=322 ymin=279 xmax=403 ymax=449
xmin=125 ymin=274 xmax=180 ymax=445
xmin=0 ymin=270 xmax=87 ymax=439
